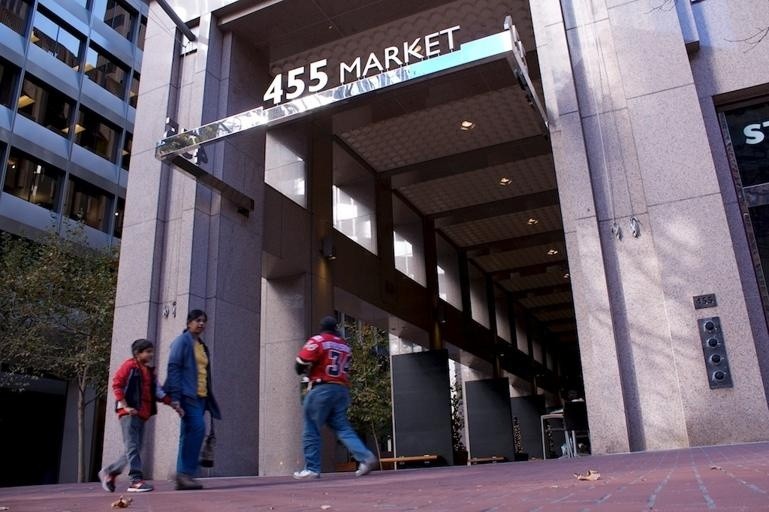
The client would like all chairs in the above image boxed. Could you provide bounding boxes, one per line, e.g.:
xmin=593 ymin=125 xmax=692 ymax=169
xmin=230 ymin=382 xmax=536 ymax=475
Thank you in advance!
xmin=562 ymin=400 xmax=589 ymax=458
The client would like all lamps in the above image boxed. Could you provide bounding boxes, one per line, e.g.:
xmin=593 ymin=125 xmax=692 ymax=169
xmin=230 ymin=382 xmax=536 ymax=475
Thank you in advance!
xmin=318 ymin=232 xmax=337 ymax=260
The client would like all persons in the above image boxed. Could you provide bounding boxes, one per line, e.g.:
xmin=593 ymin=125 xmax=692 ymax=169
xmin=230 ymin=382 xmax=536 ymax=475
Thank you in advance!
xmin=161 ymin=309 xmax=223 ymax=490
xmin=291 ymin=314 xmax=377 ymax=482
xmin=99 ymin=338 xmax=185 ymax=493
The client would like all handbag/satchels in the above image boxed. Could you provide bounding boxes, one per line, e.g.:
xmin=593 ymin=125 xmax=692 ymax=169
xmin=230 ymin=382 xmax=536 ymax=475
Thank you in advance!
xmin=197 ymin=433 xmax=217 ymax=468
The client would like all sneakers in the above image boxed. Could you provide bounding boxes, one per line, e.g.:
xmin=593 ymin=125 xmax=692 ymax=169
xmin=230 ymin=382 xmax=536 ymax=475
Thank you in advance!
xmin=355 ymin=457 xmax=379 ymax=477
xmin=175 ymin=472 xmax=203 ymax=490
xmin=97 ymin=469 xmax=115 ymax=493
xmin=292 ymin=469 xmax=321 ymax=480
xmin=127 ymin=480 xmax=154 ymax=493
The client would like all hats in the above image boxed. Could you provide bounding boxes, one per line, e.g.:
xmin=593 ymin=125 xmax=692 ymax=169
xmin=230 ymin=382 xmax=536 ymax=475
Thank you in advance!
xmin=319 ymin=316 xmax=337 ymax=333
